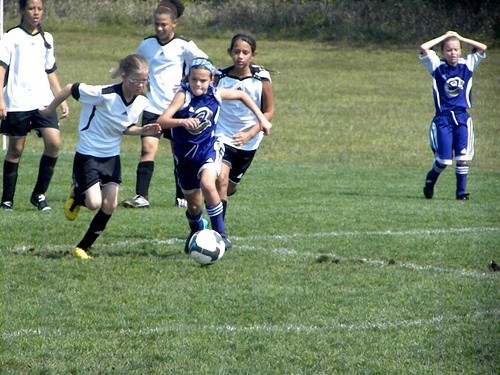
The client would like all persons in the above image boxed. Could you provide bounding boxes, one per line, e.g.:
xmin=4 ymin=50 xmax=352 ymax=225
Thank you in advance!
xmin=215 ymin=32 xmax=275 ymax=221
xmin=108 ymin=0 xmax=216 ymax=208
xmin=419 ymin=31 xmax=488 ymax=200
xmin=37 ymin=54 xmax=162 ymax=261
xmin=156 ymin=57 xmax=273 ymax=253
xmin=0 ymin=0 xmax=69 ymax=212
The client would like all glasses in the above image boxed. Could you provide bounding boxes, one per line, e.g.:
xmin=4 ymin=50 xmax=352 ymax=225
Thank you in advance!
xmin=124 ymin=75 xmax=150 ymax=86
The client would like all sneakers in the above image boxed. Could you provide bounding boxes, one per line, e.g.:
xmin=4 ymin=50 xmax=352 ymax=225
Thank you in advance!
xmin=72 ymin=246 xmax=93 ymax=260
xmin=455 ymin=189 xmax=470 ymax=200
xmin=64 ymin=184 xmax=80 ymax=221
xmin=122 ymin=195 xmax=149 ymax=208
xmin=29 ymin=192 xmax=51 ymax=211
xmin=184 ymin=231 xmax=202 ymax=253
xmin=175 ymin=198 xmax=188 ymax=208
xmin=221 ymin=233 xmax=232 ymax=249
xmin=0 ymin=201 xmax=14 ymax=211
xmin=423 ymin=171 xmax=436 ymax=199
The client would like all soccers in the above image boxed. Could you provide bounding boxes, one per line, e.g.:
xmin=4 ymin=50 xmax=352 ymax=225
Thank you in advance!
xmin=188 ymin=229 xmax=226 ymax=265
